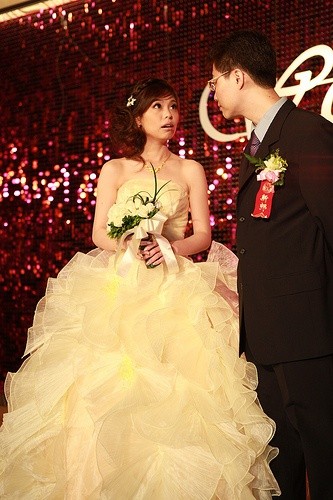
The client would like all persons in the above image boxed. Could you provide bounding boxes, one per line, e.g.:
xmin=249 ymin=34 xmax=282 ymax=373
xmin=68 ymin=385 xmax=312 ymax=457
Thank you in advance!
xmin=209 ymin=29 xmax=333 ymax=500
xmin=0 ymin=79 xmax=280 ymax=500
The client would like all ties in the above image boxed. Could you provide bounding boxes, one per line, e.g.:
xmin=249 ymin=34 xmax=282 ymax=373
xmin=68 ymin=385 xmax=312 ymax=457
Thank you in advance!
xmin=250 ymin=131 xmax=262 ymax=157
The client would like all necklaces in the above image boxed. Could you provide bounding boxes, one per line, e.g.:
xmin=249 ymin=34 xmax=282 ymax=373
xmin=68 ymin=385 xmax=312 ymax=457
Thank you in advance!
xmin=143 ymin=153 xmax=171 ymax=173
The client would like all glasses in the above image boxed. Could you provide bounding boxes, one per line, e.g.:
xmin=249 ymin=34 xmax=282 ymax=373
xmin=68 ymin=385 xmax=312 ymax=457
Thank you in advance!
xmin=207 ymin=71 xmax=230 ymax=91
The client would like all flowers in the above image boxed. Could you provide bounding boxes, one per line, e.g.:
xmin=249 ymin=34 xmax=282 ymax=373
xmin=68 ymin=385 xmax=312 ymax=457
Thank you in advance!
xmin=105 ymin=162 xmax=178 ymax=269
xmin=244 ymin=148 xmax=289 ymax=185
